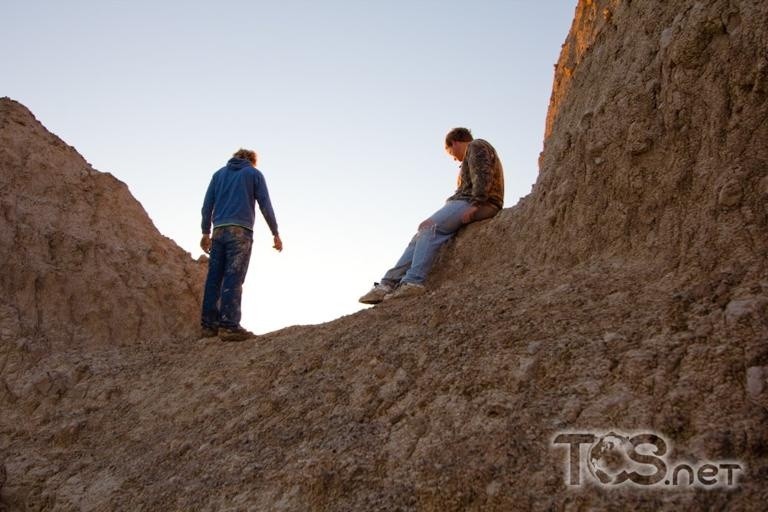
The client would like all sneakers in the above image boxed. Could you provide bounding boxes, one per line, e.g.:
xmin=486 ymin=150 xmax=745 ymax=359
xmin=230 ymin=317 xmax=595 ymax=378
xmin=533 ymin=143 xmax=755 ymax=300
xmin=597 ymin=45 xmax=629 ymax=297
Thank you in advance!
xmin=359 ymin=283 xmax=427 ymax=304
xmin=202 ymin=327 xmax=253 ymax=341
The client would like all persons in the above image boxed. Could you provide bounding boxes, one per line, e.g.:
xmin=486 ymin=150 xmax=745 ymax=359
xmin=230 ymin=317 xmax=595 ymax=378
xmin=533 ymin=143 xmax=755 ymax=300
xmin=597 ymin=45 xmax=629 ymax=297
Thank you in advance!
xmin=358 ymin=128 xmax=504 ymax=304
xmin=200 ymin=149 xmax=283 ymax=341
